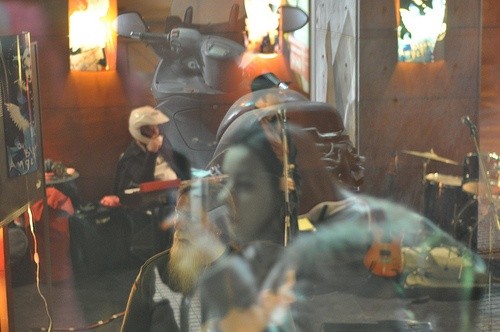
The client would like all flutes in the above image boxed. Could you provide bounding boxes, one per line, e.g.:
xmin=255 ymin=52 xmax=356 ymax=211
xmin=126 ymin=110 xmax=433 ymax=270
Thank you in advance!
xmin=280 ymin=107 xmax=297 ymax=247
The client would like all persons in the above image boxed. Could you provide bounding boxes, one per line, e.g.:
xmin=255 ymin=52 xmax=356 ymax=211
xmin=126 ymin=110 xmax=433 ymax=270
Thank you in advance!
xmin=216 ymin=122 xmax=345 ymax=332
xmin=122 ymin=178 xmax=258 ymax=332
xmin=114 ymin=106 xmax=192 ymax=262
xmin=227 ymin=73 xmax=300 ymax=247
xmin=199 ymin=239 xmax=301 ymax=332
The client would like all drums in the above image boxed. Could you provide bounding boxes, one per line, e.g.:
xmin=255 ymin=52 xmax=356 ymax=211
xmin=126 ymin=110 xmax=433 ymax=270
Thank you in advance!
xmin=462 ymin=152 xmax=500 ymax=195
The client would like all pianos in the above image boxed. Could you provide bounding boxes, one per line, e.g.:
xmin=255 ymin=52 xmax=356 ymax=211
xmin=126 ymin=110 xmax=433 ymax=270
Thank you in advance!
xmin=123 ymin=173 xmax=229 ymax=210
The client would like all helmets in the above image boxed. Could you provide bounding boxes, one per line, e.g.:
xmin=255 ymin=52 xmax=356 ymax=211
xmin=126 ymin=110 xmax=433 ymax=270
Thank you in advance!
xmin=128 ymin=105 xmax=170 ymax=149
xmin=216 ymin=87 xmax=311 ymax=147
xmin=206 ymin=100 xmax=368 ymax=207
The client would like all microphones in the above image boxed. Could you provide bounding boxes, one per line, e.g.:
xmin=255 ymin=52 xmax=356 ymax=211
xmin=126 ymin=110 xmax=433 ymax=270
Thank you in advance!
xmin=462 ymin=116 xmax=476 ymax=129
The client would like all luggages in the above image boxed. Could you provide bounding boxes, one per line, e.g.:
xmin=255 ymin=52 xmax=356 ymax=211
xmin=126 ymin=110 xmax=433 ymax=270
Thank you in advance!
xmin=69 ymin=201 xmax=130 ymax=278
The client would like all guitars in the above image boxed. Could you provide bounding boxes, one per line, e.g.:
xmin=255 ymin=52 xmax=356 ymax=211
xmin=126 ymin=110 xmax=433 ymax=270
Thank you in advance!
xmin=364 ymin=151 xmax=404 ymax=277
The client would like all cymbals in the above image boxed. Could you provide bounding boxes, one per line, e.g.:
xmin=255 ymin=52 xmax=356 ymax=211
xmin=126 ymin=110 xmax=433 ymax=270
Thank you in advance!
xmin=426 ymin=173 xmax=463 ymax=186
xmin=402 ymin=149 xmax=459 ymax=166
